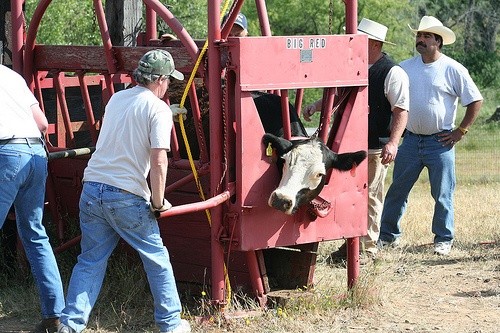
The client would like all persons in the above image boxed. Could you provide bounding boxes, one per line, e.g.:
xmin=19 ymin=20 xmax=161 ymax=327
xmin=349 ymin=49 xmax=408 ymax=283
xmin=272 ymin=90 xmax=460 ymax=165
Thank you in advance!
xmin=301 ymin=17 xmax=411 ymax=271
xmin=222 ymin=11 xmax=250 ymax=39
xmin=374 ymin=14 xmax=485 ymax=255
xmin=55 ymin=49 xmax=194 ymax=333
xmin=0 ymin=60 xmax=70 ymax=333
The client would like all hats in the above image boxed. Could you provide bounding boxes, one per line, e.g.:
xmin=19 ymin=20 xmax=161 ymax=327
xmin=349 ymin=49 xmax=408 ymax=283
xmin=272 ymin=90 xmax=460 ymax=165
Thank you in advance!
xmin=343 ymin=16 xmax=396 ymax=47
xmin=138 ymin=49 xmax=185 ymax=81
xmin=408 ymin=16 xmax=456 ymax=45
xmin=221 ymin=11 xmax=247 ymax=30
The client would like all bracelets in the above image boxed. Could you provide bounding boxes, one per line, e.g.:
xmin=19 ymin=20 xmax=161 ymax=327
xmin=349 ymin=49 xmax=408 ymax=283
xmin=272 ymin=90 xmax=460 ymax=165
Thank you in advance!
xmin=150 ymin=196 xmax=164 ymax=209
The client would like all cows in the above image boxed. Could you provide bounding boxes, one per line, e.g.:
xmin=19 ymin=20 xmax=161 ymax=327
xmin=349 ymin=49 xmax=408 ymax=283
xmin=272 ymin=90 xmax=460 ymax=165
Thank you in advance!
xmin=167 ymin=79 xmax=368 ymax=291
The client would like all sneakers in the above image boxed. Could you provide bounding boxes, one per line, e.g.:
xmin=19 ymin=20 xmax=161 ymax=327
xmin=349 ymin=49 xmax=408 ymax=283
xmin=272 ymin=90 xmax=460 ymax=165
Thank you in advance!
xmin=434 ymin=241 xmax=453 ymax=255
xmin=377 ymin=239 xmax=400 ymax=248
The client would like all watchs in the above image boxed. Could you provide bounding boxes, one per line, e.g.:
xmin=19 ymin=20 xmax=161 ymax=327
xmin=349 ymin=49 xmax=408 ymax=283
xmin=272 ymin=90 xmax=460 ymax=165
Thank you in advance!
xmin=458 ymin=126 xmax=469 ymax=135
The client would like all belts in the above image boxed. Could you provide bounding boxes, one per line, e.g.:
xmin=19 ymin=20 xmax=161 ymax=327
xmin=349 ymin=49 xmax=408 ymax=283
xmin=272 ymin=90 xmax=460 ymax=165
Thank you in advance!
xmin=0 ymin=138 xmax=44 ymax=144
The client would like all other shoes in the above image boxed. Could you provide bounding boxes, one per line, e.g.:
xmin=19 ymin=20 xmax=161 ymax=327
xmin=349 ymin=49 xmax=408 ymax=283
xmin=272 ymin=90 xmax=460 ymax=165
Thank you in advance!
xmin=42 ymin=318 xmax=61 ymax=333
xmin=58 ymin=323 xmax=74 ymax=333
xmin=325 ymin=245 xmax=373 ymax=266
xmin=160 ymin=319 xmax=191 ymax=333
